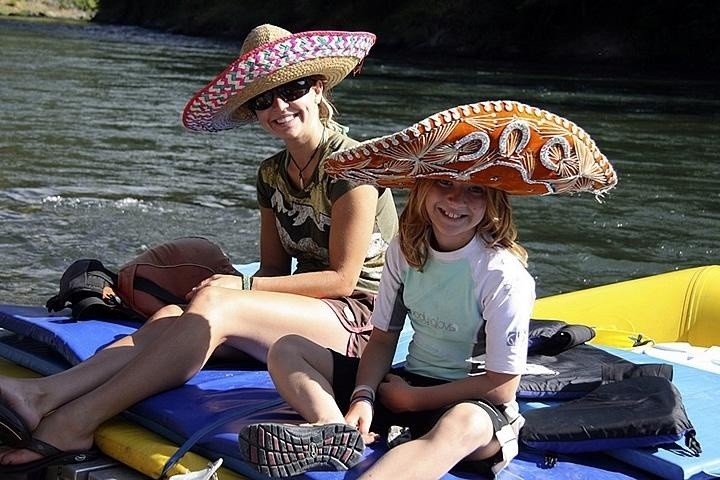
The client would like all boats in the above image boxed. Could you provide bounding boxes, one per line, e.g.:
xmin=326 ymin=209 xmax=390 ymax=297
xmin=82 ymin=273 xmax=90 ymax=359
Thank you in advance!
xmin=1 ymin=262 xmax=720 ymax=479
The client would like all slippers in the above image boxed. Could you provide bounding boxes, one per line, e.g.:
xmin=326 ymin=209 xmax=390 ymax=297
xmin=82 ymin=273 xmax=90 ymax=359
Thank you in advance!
xmin=0 ymin=402 xmax=98 ymax=474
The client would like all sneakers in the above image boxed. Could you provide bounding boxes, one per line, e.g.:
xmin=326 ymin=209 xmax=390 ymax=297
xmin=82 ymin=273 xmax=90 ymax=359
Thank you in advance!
xmin=237 ymin=423 xmax=364 ymax=478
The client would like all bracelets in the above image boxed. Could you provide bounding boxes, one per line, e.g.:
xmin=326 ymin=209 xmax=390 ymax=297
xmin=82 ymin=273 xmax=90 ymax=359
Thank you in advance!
xmin=349 ymin=395 xmax=374 ymax=408
xmin=242 ymin=274 xmax=255 ymax=289
xmin=350 ymin=384 xmax=376 ymax=405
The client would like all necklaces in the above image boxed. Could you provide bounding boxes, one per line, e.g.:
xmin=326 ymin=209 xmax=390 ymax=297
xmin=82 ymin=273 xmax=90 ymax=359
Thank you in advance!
xmin=288 ymin=144 xmax=318 ymax=191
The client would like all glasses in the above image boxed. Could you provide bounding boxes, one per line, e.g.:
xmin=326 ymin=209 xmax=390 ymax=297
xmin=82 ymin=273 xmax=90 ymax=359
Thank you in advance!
xmin=249 ymin=79 xmax=315 ymax=110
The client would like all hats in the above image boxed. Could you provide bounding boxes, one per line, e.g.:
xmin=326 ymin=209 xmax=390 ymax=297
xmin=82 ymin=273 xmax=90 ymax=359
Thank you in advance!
xmin=322 ymin=101 xmax=618 ymax=195
xmin=183 ymin=24 xmax=377 ymax=132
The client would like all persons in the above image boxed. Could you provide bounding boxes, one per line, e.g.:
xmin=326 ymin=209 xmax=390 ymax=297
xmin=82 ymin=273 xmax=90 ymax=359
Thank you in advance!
xmin=237 ymin=100 xmax=618 ymax=480
xmin=0 ymin=27 xmax=398 ymax=472
